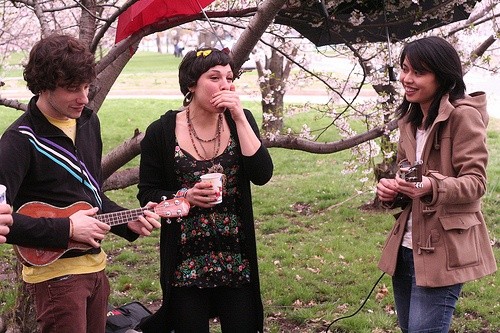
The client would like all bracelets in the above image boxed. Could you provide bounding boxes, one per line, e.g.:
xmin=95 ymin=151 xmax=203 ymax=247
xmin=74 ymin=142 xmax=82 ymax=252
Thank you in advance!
xmin=69 ymin=219 xmax=74 ymax=240
xmin=175 ymin=187 xmax=188 ymax=199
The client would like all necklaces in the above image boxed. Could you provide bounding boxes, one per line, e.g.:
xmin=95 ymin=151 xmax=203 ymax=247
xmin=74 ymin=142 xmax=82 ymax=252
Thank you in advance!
xmin=186 ymin=104 xmax=223 ymax=161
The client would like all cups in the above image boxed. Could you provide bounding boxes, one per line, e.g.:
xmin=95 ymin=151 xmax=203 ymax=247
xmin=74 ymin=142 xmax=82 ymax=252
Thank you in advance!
xmin=200 ymin=173 xmax=223 ymax=204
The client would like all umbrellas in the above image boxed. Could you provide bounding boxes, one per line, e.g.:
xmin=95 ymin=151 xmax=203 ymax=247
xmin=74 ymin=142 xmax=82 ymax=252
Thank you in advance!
xmin=116 ymin=0 xmax=232 ymax=55
xmin=273 ymin=0 xmax=478 ymax=82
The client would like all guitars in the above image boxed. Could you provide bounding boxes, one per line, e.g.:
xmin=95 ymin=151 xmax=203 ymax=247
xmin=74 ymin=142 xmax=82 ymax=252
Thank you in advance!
xmin=12 ymin=194 xmax=190 ymax=269
xmin=398 ymin=159 xmax=423 ymax=182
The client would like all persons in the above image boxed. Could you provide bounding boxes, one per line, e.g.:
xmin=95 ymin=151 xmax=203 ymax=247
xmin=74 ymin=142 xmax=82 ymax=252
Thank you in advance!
xmin=155 ymin=33 xmax=161 ymax=53
xmin=137 ymin=47 xmax=273 ymax=333
xmin=374 ymin=36 xmax=498 ymax=333
xmin=173 ymin=38 xmax=185 ymax=57
xmin=0 ymin=32 xmax=161 ymax=333
xmin=0 ymin=204 xmax=13 ymax=243
